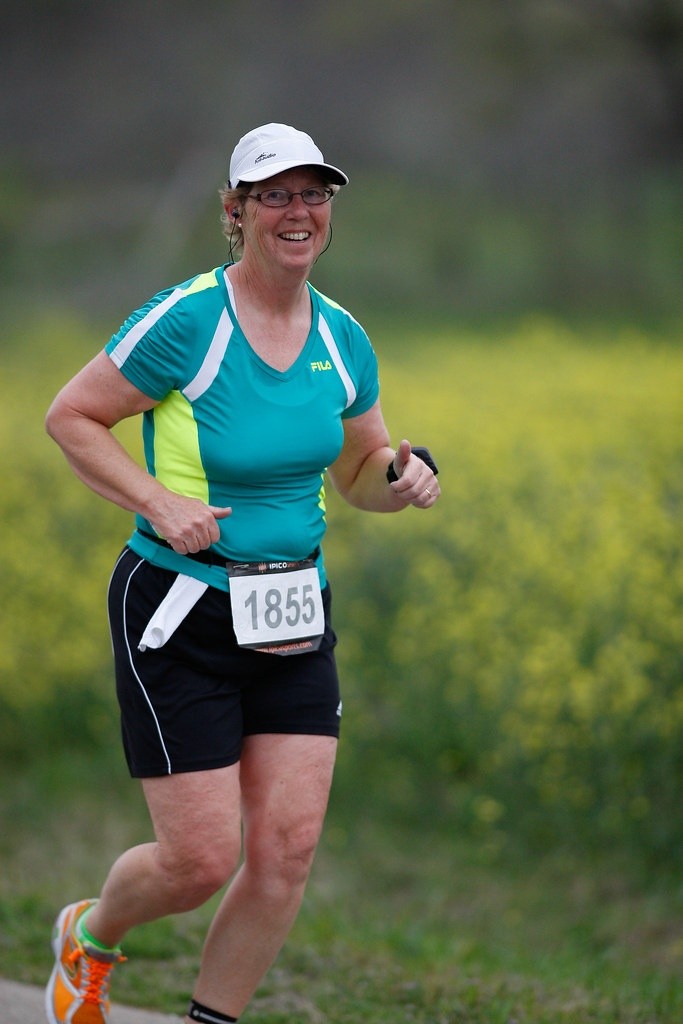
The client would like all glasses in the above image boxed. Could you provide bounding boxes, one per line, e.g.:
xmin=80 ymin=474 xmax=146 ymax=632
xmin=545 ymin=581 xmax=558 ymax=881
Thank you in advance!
xmin=237 ymin=185 xmax=333 ymax=208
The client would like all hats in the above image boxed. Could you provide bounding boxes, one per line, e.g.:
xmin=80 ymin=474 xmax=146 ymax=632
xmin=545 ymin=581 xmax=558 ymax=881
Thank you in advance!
xmin=229 ymin=123 xmax=349 ymax=191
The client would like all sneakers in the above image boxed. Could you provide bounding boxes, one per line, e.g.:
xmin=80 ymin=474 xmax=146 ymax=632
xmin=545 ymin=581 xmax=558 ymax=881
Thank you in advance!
xmin=45 ymin=898 xmax=128 ymax=1024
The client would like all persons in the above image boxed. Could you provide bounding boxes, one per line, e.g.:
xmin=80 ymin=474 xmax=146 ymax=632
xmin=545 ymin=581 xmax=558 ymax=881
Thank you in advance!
xmin=44 ymin=123 xmax=440 ymax=1024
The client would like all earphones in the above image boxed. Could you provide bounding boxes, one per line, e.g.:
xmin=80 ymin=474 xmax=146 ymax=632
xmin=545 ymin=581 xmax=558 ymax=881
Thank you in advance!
xmin=231 ymin=207 xmax=240 ymax=217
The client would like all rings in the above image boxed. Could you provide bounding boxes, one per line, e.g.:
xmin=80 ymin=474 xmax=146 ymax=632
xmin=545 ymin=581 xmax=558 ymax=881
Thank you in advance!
xmin=426 ymin=489 xmax=432 ymax=499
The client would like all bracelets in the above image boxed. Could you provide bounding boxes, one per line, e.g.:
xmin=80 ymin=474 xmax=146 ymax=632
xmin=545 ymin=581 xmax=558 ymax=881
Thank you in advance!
xmin=386 ymin=446 xmax=439 ymax=493
